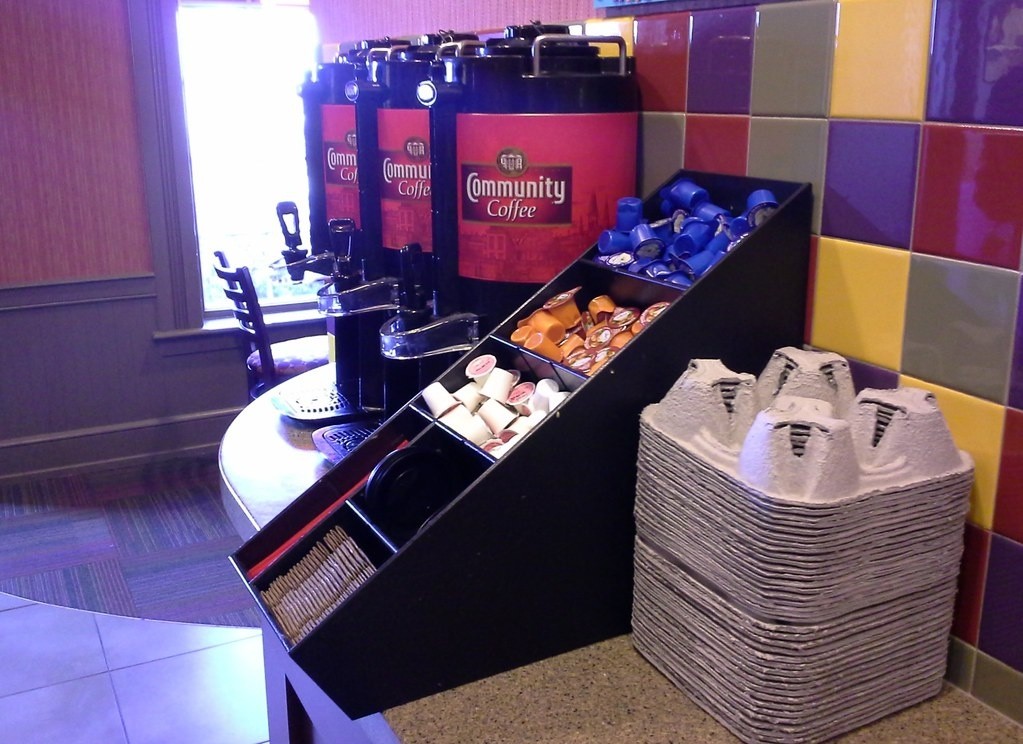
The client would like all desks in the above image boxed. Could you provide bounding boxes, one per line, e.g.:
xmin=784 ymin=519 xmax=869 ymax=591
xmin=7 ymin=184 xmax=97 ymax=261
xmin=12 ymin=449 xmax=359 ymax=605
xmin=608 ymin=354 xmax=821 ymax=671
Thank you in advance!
xmin=216 ymin=360 xmax=1023 ymax=744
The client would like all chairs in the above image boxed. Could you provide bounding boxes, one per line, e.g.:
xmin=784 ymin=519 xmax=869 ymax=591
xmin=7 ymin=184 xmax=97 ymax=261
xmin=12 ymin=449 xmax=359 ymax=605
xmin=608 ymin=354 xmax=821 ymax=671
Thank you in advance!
xmin=211 ymin=250 xmax=328 ymax=402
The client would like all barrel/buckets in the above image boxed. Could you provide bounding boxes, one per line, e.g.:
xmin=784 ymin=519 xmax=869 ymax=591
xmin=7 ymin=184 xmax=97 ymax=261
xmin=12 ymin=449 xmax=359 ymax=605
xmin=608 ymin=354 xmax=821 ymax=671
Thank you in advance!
xmin=303 ymin=23 xmax=641 ymax=429
